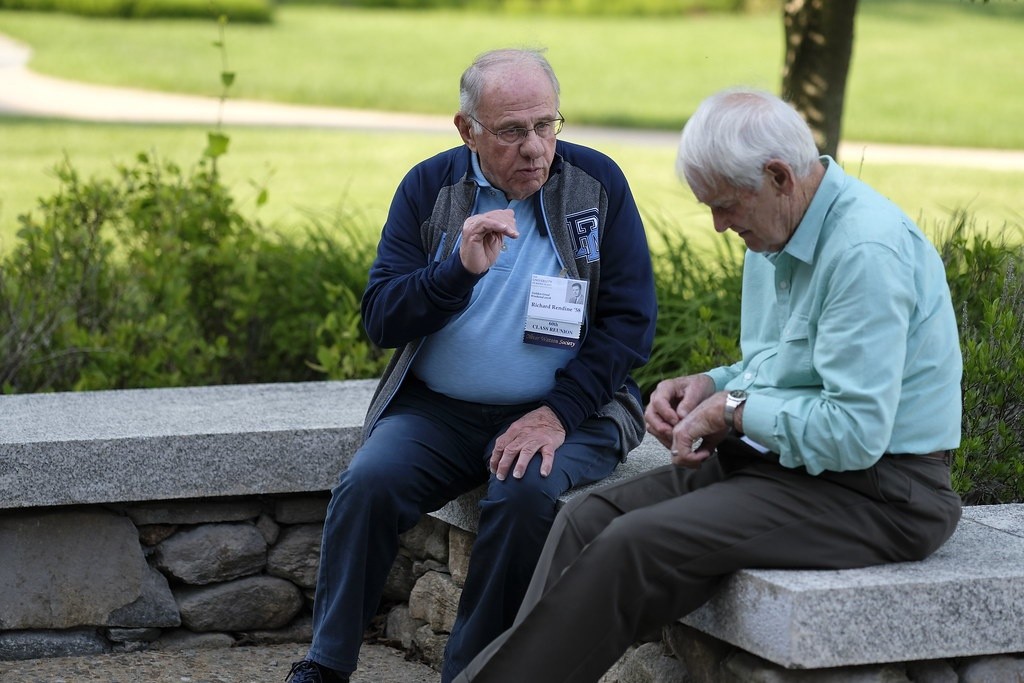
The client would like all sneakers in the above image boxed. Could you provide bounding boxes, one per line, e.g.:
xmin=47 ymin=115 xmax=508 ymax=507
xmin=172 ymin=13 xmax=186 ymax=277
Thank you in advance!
xmin=285 ymin=658 xmax=350 ymax=683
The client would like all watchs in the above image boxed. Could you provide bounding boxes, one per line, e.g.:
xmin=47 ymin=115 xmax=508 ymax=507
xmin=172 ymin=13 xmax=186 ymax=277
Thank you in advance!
xmin=724 ymin=389 xmax=748 ymax=426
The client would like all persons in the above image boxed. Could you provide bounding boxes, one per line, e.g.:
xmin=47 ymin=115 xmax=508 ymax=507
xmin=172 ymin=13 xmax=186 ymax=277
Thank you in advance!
xmin=282 ymin=48 xmax=661 ymax=683
xmin=568 ymin=283 xmax=584 ymax=304
xmin=446 ymin=85 xmax=971 ymax=683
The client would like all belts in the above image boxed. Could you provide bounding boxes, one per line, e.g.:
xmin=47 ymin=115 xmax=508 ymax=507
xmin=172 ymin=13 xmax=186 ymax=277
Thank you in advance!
xmin=907 ymin=448 xmax=951 ymax=461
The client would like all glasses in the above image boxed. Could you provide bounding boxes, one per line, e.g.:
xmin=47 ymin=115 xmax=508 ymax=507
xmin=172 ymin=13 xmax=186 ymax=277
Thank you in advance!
xmin=469 ymin=108 xmax=565 ymax=146
